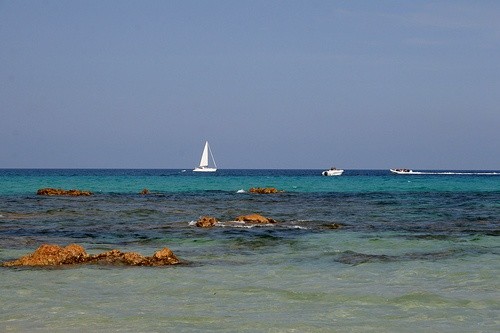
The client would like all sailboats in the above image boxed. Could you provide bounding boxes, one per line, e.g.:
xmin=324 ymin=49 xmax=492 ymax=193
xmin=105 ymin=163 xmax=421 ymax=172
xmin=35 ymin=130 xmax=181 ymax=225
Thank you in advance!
xmin=193 ymin=140 xmax=217 ymax=172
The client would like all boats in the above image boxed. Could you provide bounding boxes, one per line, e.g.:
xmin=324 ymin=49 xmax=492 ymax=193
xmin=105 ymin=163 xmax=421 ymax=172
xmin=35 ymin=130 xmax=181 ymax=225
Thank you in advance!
xmin=322 ymin=168 xmax=343 ymax=176
xmin=390 ymin=167 xmax=499 ymax=176
